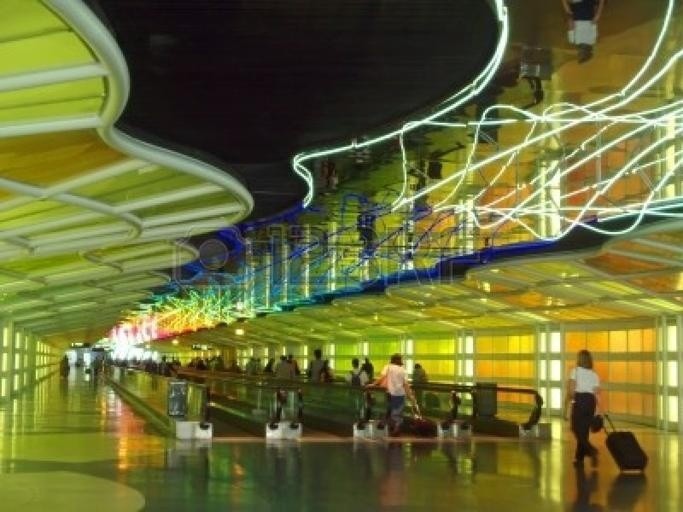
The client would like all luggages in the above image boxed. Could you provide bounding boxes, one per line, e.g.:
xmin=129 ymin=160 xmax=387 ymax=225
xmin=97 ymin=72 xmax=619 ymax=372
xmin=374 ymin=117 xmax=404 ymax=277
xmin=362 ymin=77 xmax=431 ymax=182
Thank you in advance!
xmin=598 ymin=414 xmax=649 ymax=472
xmin=408 ymin=405 xmax=438 ymax=438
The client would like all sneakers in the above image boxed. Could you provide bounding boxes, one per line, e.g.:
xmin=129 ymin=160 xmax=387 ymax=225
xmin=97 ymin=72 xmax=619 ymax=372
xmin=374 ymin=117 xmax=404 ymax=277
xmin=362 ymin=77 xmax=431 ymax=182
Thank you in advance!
xmin=573 ymin=460 xmax=584 ymax=468
xmin=591 ymin=447 xmax=599 ymax=468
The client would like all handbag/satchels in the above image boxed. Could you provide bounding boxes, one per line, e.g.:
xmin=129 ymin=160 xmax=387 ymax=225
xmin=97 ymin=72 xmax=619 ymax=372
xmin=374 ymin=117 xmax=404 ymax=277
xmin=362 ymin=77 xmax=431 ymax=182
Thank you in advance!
xmin=318 ymin=362 xmax=328 ymax=383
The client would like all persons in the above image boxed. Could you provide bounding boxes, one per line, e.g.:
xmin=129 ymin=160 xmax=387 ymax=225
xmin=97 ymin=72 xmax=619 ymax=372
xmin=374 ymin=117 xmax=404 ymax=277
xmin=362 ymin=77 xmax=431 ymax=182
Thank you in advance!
xmin=109 ymin=355 xmax=181 ymax=381
xmin=345 ymin=353 xmax=426 ymax=435
xmin=564 ymin=348 xmax=600 ymax=469
xmin=277 ymin=350 xmax=333 ymax=382
xmin=60 ymin=347 xmax=105 ymax=391
xmin=187 ymin=354 xmax=275 ymax=373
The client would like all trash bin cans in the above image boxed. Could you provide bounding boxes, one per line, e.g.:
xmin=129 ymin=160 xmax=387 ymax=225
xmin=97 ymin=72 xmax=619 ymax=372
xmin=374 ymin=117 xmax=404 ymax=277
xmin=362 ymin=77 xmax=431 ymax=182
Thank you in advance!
xmin=168 ymin=382 xmax=187 ymax=416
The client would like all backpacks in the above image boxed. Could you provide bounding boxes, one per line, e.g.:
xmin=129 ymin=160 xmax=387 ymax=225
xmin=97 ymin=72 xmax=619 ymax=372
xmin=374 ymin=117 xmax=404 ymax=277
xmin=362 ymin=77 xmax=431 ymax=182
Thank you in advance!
xmin=351 ymin=369 xmax=363 ymax=386
